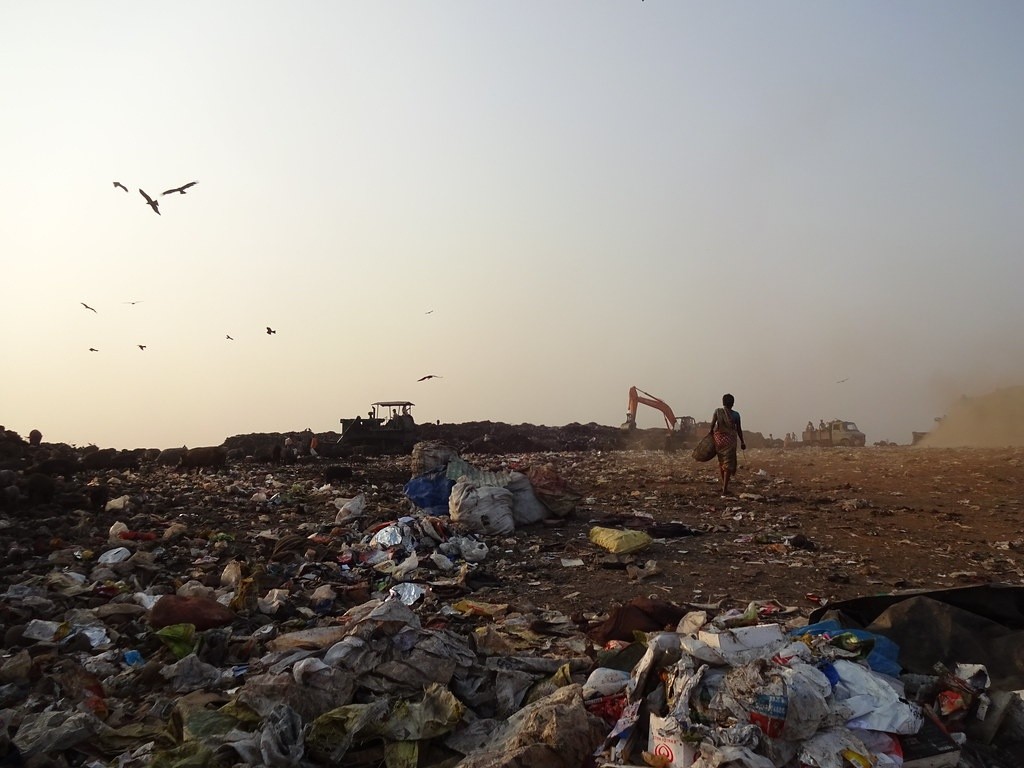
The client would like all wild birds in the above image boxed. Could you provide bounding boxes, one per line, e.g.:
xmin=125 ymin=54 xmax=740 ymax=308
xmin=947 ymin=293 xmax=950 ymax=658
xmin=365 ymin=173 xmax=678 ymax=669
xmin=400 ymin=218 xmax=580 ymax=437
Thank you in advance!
xmin=425 ymin=311 xmax=432 ymax=315
xmin=138 ymin=345 xmax=147 ymax=350
xmin=90 ymin=348 xmax=98 ymax=351
xmin=226 ymin=335 xmax=234 ymax=340
xmin=124 ymin=301 xmax=143 ymax=305
xmin=418 ymin=375 xmax=443 ymax=381
xmin=266 ymin=327 xmax=276 ymax=334
xmin=163 ymin=181 xmax=196 ymax=196
xmin=81 ymin=303 xmax=96 ymax=313
xmin=113 ymin=182 xmax=128 ymax=192
xmin=138 ymin=189 xmax=161 ymax=216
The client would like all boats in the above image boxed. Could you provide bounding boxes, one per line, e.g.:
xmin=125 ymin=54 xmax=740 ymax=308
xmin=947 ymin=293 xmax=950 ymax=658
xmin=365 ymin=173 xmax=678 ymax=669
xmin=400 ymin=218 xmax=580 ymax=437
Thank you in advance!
xmin=340 ymin=402 xmax=421 ymax=441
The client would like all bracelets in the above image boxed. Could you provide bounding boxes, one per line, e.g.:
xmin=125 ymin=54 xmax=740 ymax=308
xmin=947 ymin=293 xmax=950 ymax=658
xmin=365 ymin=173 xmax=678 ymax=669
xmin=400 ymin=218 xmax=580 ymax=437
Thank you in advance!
xmin=741 ymin=443 xmax=744 ymax=444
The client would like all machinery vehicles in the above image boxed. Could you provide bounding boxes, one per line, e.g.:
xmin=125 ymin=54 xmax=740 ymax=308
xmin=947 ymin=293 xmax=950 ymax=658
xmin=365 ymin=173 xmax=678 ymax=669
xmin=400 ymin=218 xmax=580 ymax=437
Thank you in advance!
xmin=621 ymin=386 xmax=714 ymax=453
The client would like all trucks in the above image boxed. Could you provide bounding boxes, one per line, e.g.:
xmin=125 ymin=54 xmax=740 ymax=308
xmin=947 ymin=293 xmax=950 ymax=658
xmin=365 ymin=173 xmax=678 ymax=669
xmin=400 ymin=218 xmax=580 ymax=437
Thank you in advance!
xmin=801 ymin=421 xmax=866 ymax=448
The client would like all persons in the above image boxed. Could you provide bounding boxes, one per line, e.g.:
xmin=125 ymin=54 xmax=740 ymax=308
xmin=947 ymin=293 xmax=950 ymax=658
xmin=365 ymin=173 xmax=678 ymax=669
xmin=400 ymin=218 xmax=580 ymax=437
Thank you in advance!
xmin=807 ymin=421 xmax=814 ymax=430
xmin=402 ymin=405 xmax=410 ymax=414
xmin=310 ymin=436 xmax=320 ymax=456
xmin=390 ymin=409 xmax=398 ymax=419
xmin=792 ymin=432 xmax=796 ymax=441
xmin=272 ymin=444 xmax=281 ymax=467
xmin=666 ymin=434 xmax=671 ymax=454
xmin=770 ymin=433 xmax=774 ymax=447
xmin=819 ymin=419 xmax=825 ymax=430
xmin=708 ymin=394 xmax=746 ymax=495
